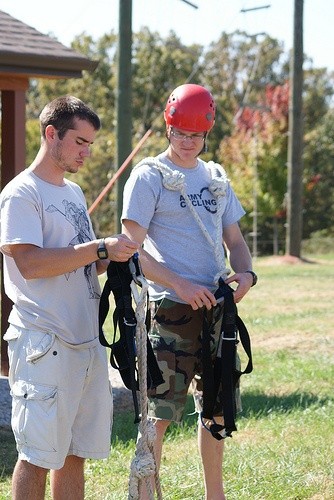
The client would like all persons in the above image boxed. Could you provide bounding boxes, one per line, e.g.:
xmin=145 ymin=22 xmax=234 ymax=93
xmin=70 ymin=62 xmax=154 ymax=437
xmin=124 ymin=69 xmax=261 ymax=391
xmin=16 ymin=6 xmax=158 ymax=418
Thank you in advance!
xmin=121 ymin=83 xmax=258 ymax=500
xmin=0 ymin=96 xmax=140 ymax=500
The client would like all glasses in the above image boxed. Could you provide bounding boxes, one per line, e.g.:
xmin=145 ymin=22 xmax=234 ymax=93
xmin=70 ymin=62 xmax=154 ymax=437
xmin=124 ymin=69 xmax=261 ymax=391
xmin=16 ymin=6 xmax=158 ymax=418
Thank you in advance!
xmin=169 ymin=129 xmax=205 ymax=143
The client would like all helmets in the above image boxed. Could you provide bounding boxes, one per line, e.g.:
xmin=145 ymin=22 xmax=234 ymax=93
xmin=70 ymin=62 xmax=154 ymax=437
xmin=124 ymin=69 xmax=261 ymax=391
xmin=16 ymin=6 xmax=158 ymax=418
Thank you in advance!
xmin=164 ymin=84 xmax=216 ymax=132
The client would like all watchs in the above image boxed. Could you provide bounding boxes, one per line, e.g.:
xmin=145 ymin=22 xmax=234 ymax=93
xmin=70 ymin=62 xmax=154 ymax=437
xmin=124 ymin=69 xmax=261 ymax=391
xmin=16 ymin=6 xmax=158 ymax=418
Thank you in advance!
xmin=97 ymin=238 xmax=108 ymax=259
xmin=245 ymin=270 xmax=257 ymax=287
